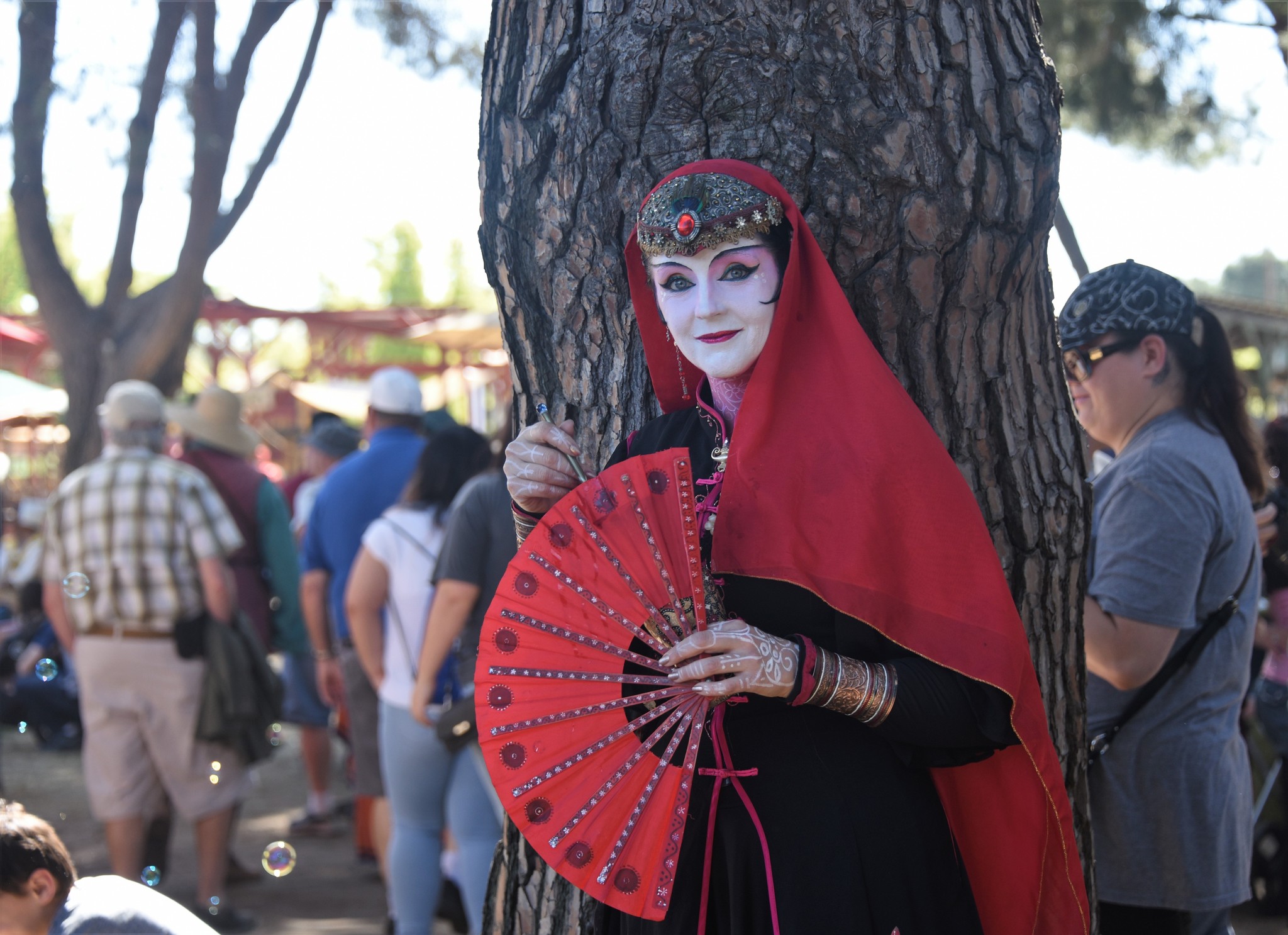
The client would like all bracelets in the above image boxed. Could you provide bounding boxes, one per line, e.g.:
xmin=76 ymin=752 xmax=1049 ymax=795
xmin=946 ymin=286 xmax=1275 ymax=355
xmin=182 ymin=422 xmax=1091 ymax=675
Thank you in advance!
xmin=802 ymin=644 xmax=898 ymax=727
xmin=315 ymin=647 xmax=333 ymax=661
xmin=511 ymin=503 xmax=538 ymax=551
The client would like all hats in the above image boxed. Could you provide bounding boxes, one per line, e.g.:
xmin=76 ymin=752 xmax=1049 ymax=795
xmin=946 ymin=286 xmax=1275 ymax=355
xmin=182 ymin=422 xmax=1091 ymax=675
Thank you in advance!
xmin=1057 ymin=259 xmax=1193 ymax=341
xmin=366 ymin=367 xmax=421 ymax=416
xmin=169 ymin=387 xmax=256 ymax=457
xmin=302 ymin=412 xmax=361 ymax=457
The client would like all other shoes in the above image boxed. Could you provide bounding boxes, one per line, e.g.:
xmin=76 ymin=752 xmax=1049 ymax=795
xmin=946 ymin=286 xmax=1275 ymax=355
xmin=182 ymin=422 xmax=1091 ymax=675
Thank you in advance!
xmin=290 ymin=810 xmax=345 ymax=839
xmin=228 ymin=855 xmax=263 ymax=884
xmin=198 ymin=906 xmax=258 ymax=935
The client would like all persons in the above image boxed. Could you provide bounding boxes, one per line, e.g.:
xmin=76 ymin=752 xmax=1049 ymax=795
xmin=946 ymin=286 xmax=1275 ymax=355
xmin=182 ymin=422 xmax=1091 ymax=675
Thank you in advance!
xmin=40 ymin=378 xmax=257 ymax=935
xmin=289 ymin=417 xmax=359 ymax=836
xmin=345 ymin=423 xmax=504 ymax=935
xmin=175 ymin=382 xmax=301 ymax=886
xmin=414 ymin=470 xmax=517 ymax=829
xmin=0 ymin=798 xmax=221 ymax=935
xmin=1057 ymin=259 xmax=1262 ymax=935
xmin=504 ymin=159 xmax=1090 ymax=935
xmin=301 ymin=366 xmax=428 ymax=876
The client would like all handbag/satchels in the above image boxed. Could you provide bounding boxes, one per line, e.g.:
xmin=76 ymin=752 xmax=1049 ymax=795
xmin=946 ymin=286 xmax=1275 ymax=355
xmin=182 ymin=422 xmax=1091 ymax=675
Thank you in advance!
xmin=436 ymin=694 xmax=480 ymax=752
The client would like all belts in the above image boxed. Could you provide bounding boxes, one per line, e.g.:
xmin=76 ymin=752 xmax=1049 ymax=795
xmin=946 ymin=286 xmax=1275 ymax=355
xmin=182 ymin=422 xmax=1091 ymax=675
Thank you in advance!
xmin=84 ymin=625 xmax=175 ymax=638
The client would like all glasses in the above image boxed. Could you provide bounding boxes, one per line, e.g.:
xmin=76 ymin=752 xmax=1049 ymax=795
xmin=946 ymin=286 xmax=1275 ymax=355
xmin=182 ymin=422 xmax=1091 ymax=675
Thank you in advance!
xmin=1060 ymin=334 xmax=1142 ymax=382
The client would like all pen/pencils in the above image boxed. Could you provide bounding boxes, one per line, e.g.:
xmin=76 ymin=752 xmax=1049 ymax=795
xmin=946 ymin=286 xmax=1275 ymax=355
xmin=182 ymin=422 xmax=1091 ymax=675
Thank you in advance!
xmin=537 ymin=403 xmax=587 ymax=483
